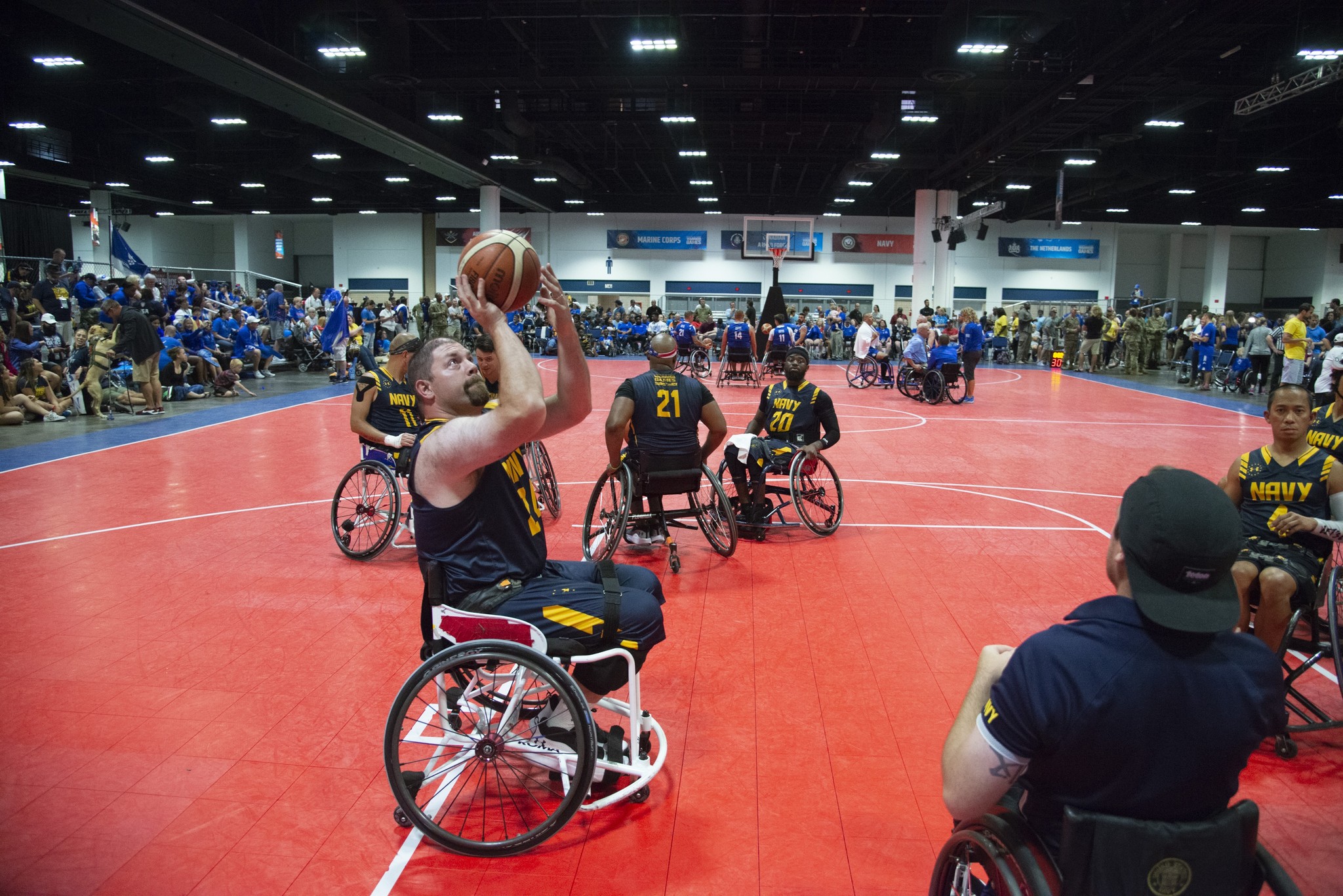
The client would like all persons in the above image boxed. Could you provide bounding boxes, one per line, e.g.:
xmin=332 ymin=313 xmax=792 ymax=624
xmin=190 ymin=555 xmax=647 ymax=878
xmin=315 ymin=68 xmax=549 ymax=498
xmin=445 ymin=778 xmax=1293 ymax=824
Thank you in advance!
xmin=0 ymin=247 xmax=1343 ymax=426
xmin=409 ymin=264 xmax=664 ymax=766
xmin=350 ymin=334 xmax=421 ymax=536
xmin=1219 ymin=349 xmax=1343 ymax=490
xmin=724 ymin=346 xmax=840 ymax=521
xmin=1224 ymin=385 xmax=1343 ymax=655
xmin=603 ymin=333 xmax=726 ymax=544
xmin=464 ymin=337 xmax=529 ymax=453
xmin=941 ymin=465 xmax=1285 ymax=851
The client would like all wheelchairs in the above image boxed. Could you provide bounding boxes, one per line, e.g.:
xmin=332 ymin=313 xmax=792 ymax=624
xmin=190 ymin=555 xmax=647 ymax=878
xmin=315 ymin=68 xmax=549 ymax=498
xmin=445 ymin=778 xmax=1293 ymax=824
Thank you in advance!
xmin=346 ymin=335 xmax=368 ymax=376
xmin=896 ymin=350 xmax=968 ymax=405
xmin=928 ymin=785 xmax=1306 ymax=896
xmin=716 ymin=347 xmax=761 ymax=388
xmin=583 ymin=446 xmax=738 ymax=572
xmin=1171 ymin=347 xmax=1256 ymax=393
xmin=673 ymin=342 xmax=712 ymax=378
xmin=710 ymin=432 xmax=844 ymax=536
xmin=383 ymin=557 xmax=668 ymax=857
xmin=758 ymin=345 xmax=788 ymax=380
xmin=846 ymin=341 xmax=895 ymax=389
xmin=520 ymin=441 xmax=562 ymax=518
xmin=1235 ymin=540 xmax=1342 ymax=757
xmin=331 ymin=435 xmax=416 ymax=561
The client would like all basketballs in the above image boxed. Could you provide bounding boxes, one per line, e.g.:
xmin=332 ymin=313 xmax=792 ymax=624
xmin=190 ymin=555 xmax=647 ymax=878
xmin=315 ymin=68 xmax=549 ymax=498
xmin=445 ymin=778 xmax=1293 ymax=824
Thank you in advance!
xmin=761 ymin=323 xmax=772 ymax=334
xmin=457 ymin=229 xmax=541 ymax=314
xmin=702 ymin=338 xmax=712 ymax=346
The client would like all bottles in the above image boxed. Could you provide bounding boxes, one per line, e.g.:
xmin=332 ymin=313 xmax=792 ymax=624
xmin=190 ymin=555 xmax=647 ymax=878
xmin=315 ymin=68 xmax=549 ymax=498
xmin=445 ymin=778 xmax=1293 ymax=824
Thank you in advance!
xmin=70 ymin=295 xmax=78 ymax=312
xmin=41 ymin=345 xmax=49 ymax=363
xmin=75 ymin=305 xmax=80 ymax=323
xmin=1307 ymin=343 xmax=1313 ymax=355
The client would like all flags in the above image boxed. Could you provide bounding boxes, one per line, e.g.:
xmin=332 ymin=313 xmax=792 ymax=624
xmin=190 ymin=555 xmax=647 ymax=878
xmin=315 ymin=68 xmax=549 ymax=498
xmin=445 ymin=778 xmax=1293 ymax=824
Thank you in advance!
xmin=110 ymin=221 xmax=149 ymax=280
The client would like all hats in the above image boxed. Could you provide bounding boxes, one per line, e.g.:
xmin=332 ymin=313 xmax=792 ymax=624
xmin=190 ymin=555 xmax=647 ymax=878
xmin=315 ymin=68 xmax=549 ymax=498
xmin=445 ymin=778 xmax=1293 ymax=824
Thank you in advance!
xmin=41 ymin=313 xmax=58 ymax=324
xmin=47 ymin=264 xmax=61 ymax=274
xmin=1334 ymin=333 xmax=1343 ymax=342
xmin=1050 ymin=308 xmax=1057 ymax=313
xmin=219 ymin=283 xmax=229 ymax=287
xmin=341 ymin=287 xmax=348 ymax=294
xmin=246 ymin=316 xmax=261 ymax=324
xmin=614 ymin=300 xmax=621 ymax=305
xmin=945 ymin=319 xmax=953 ymax=324
xmin=400 ymin=296 xmax=408 ymax=300
xmin=444 ymin=293 xmax=450 ymax=297
xmin=897 ymin=307 xmax=903 ymax=311
xmin=1253 ymin=313 xmax=1263 ymax=317
xmin=586 ymin=305 xmax=591 ymax=309
xmin=573 ymin=301 xmax=580 ymax=307
xmin=1119 ymin=468 xmax=1245 ymax=634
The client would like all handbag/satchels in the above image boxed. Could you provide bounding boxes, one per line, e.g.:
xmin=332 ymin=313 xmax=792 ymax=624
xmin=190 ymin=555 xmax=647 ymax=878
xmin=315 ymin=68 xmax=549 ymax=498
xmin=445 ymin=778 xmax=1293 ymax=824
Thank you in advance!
xmin=996 ymin=352 xmax=1010 ymax=364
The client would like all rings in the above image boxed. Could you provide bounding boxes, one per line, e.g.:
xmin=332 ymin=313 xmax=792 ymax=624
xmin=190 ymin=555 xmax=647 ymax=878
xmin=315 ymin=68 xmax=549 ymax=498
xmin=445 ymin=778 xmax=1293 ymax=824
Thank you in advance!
xmin=552 ymin=293 xmax=563 ymax=300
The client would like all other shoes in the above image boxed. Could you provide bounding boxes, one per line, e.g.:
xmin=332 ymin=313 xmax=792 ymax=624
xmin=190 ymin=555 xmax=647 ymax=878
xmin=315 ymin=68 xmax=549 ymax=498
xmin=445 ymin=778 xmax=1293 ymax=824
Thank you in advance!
xmin=1074 ymin=368 xmax=1093 ymax=373
xmin=162 ymin=386 xmax=174 ymax=401
xmin=1249 ymin=389 xmax=1266 ymax=395
xmin=1194 ymin=387 xmax=1211 ymax=390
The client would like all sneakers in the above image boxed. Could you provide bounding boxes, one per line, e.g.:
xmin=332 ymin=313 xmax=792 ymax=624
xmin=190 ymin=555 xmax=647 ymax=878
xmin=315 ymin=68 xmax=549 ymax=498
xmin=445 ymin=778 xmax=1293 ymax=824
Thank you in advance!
xmin=732 ymin=374 xmax=745 ymax=380
xmin=43 ymin=411 xmax=66 ymax=422
xmin=692 ymin=364 xmax=704 ymax=371
xmin=958 ymin=395 xmax=974 ymax=403
xmin=329 ymin=377 xmax=349 ymax=385
xmin=135 ymin=407 xmax=165 ymax=415
xmin=523 ymin=691 xmax=629 ymax=783
xmin=774 ymin=369 xmax=785 ymax=375
xmin=253 ymin=370 xmax=276 ymax=378
xmin=735 ymin=503 xmax=770 ymax=540
xmin=622 ymin=522 xmax=665 ymax=546
xmin=61 ymin=410 xmax=72 ymax=417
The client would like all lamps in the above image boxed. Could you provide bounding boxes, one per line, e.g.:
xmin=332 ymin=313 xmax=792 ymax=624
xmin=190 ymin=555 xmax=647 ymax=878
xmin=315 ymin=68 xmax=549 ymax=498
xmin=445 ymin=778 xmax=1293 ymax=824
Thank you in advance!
xmin=81 ymin=215 xmax=131 ymax=232
xmin=931 ymin=216 xmax=988 ymax=250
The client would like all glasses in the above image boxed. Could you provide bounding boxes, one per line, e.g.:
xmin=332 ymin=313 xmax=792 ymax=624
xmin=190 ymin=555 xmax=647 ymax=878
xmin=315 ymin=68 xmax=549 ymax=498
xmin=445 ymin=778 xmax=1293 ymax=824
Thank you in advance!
xmin=43 ymin=321 xmax=56 ymax=326
xmin=22 ymin=267 xmax=33 ymax=271
xmin=586 ymin=306 xmax=589 ymax=307
xmin=105 ymin=306 xmax=113 ymax=314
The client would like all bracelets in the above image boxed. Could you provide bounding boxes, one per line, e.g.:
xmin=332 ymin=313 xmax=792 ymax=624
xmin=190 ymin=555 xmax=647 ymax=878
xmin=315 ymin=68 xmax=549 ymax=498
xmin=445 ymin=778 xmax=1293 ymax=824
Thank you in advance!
xmin=609 ymin=461 xmax=622 ymax=469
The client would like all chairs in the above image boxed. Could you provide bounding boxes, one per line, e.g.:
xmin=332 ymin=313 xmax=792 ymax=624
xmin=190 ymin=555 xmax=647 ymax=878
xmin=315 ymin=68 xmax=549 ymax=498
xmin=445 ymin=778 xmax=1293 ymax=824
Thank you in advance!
xmin=990 ymin=336 xmax=1010 ymax=364
xmin=1168 ymin=347 xmax=1234 ymax=384
xmin=522 ymin=325 xmax=601 ymax=357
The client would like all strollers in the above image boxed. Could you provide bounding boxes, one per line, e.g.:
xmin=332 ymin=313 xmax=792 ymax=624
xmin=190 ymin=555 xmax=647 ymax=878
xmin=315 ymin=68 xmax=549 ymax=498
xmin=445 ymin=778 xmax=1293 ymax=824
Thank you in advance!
xmin=289 ymin=325 xmax=337 ymax=373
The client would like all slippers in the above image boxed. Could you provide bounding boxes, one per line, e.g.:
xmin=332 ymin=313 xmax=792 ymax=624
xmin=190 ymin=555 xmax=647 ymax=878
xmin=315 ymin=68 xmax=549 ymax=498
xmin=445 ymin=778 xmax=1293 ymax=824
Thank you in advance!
xmin=200 ymin=391 xmax=211 ymax=398
